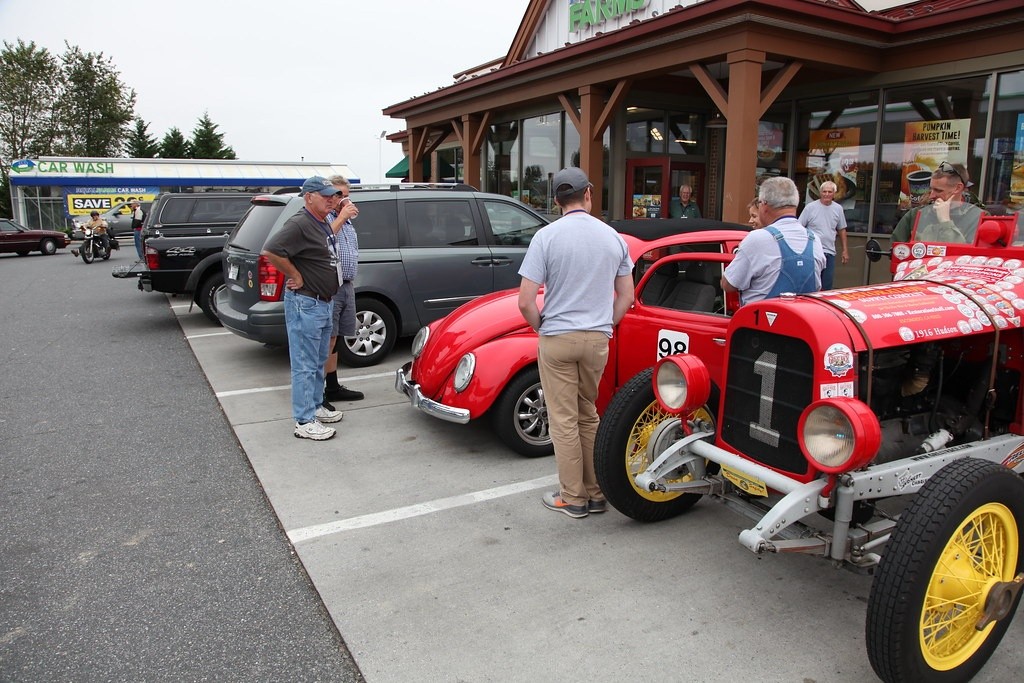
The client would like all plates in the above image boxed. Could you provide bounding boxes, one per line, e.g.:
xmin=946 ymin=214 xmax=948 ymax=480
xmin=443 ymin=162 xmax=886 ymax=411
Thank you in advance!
xmin=809 ymin=177 xmax=856 ymax=203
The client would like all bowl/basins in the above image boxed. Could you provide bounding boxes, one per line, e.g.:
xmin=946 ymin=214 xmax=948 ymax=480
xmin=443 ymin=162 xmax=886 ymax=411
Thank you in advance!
xmin=757 ymin=148 xmax=776 ymax=162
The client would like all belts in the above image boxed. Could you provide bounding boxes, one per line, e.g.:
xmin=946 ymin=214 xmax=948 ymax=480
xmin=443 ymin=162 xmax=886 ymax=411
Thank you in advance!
xmin=343 ymin=279 xmax=351 ymax=284
xmin=288 ymin=285 xmax=332 ymax=302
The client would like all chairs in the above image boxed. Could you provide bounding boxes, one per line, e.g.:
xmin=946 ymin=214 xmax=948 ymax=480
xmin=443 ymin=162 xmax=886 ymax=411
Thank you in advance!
xmin=441 ymin=218 xmax=475 ymax=247
xmin=639 ymin=263 xmax=716 ymax=314
xmin=415 ymin=216 xmax=443 ymax=248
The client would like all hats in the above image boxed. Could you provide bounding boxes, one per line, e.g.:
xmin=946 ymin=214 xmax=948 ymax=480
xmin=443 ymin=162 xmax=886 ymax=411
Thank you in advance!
xmin=553 ymin=167 xmax=594 ymax=196
xmin=298 ymin=176 xmax=342 ymax=196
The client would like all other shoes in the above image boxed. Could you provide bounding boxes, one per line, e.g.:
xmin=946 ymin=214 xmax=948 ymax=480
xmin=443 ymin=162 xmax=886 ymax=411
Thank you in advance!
xmin=71 ymin=250 xmax=79 ymax=256
xmin=106 ymin=246 xmax=111 ymax=253
xmin=136 ymin=259 xmax=145 ymax=262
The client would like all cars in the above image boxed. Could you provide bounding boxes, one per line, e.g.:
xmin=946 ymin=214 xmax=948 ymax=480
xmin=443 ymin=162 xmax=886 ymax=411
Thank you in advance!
xmin=593 ymin=202 xmax=1024 ymax=683
xmin=0 ymin=218 xmax=72 ymax=256
xmin=113 ymin=192 xmax=269 ymax=327
xmin=72 ymin=201 xmax=153 ymax=239
xmin=394 ymin=216 xmax=753 ymax=458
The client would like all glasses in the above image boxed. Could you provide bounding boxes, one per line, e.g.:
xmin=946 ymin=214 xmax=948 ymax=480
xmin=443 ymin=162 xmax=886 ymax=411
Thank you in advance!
xmin=754 ymin=198 xmax=767 ymax=209
xmin=939 ymin=161 xmax=965 ymax=187
xmin=583 ymin=188 xmax=593 ymax=198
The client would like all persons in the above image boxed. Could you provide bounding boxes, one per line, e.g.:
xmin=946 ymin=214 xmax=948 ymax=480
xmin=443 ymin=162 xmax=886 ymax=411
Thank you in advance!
xmin=517 ymin=167 xmax=637 ymax=517
xmin=263 ymin=176 xmax=343 ymax=440
xmin=670 ymin=184 xmax=702 ymax=218
xmin=71 ymin=210 xmax=111 ymax=257
xmin=321 ymin=174 xmax=365 ymax=410
xmin=888 ymin=162 xmax=991 ymax=253
xmin=130 ymin=200 xmax=146 ymax=263
xmin=797 ymin=181 xmax=848 ymax=290
xmin=747 ymin=198 xmax=764 ymax=231
xmin=721 ymin=176 xmax=827 ymax=307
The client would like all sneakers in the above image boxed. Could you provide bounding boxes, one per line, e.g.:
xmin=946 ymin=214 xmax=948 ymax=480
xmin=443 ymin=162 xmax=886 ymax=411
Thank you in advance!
xmin=588 ymin=499 xmax=606 ymax=512
xmin=542 ymin=491 xmax=588 ymax=517
xmin=325 ymin=385 xmax=364 ymax=401
xmin=294 ymin=419 xmax=337 ymax=440
xmin=322 ymin=400 xmax=335 ymax=410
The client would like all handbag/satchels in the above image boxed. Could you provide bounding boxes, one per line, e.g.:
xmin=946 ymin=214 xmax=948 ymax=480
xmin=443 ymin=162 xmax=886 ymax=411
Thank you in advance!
xmin=315 ymin=406 xmax=343 ymax=422
xmin=109 ymin=237 xmax=118 ymax=249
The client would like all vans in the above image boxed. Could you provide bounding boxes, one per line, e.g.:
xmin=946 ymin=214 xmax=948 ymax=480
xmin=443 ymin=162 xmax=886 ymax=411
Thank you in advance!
xmin=214 ymin=183 xmax=551 ymax=369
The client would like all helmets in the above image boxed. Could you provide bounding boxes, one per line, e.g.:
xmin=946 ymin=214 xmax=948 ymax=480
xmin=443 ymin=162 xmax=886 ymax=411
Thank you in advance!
xmin=90 ymin=211 xmax=99 ymax=217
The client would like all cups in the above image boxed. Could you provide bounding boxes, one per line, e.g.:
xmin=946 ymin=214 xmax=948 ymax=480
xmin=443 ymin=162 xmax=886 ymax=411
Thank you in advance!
xmin=907 ymin=170 xmax=933 ymax=209
xmin=339 ymin=197 xmax=359 ymax=219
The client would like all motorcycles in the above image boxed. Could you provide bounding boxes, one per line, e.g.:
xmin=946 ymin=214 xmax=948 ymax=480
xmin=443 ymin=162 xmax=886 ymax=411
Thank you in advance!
xmin=78 ymin=225 xmax=113 ymax=264
